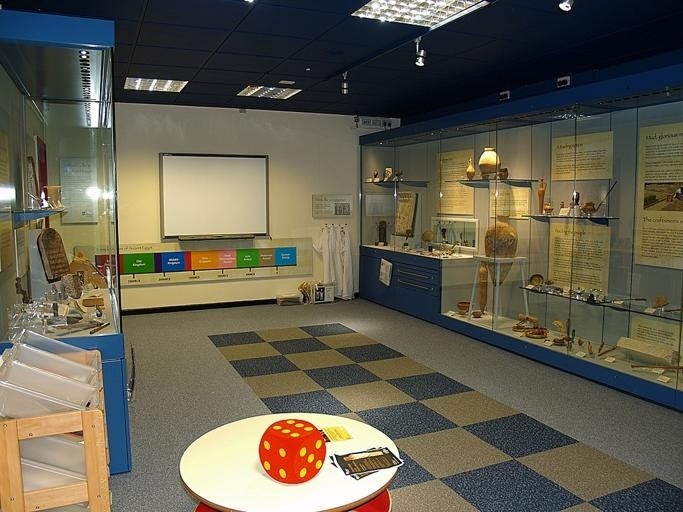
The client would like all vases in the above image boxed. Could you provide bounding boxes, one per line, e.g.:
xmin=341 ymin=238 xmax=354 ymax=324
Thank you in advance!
xmin=479 ymin=148 xmax=501 ymax=178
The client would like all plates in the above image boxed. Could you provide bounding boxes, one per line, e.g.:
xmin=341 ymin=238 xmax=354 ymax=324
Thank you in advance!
xmin=530 ymin=274 xmax=544 ymax=286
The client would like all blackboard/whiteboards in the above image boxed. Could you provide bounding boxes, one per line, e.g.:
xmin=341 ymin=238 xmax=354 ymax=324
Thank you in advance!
xmin=159 ymin=152 xmax=269 ymax=241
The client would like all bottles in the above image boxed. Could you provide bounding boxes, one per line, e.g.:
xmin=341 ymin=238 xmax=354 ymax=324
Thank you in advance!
xmin=316 ymin=288 xmax=324 ymax=301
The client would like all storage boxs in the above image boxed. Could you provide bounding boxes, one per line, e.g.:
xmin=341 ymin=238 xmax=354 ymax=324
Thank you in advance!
xmin=0 ymin=330 xmax=99 ymax=512
xmin=297 ymin=281 xmax=335 ymax=303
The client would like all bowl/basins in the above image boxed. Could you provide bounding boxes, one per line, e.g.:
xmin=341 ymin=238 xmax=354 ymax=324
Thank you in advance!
xmin=473 ymin=312 xmax=483 ymax=317
xmin=458 ymin=301 xmax=473 ymax=311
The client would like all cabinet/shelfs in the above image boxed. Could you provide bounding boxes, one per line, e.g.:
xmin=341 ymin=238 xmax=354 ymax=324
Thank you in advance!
xmin=355 ymin=48 xmax=682 ymax=412
xmin=0 ymin=9 xmax=136 ymax=476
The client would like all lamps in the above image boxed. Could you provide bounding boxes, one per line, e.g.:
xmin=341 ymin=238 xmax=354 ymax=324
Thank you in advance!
xmin=317 ymin=0 xmax=576 ymax=93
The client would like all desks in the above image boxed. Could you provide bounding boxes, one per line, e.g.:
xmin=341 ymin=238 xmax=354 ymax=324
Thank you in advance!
xmin=178 ymin=413 xmax=399 ymax=512
